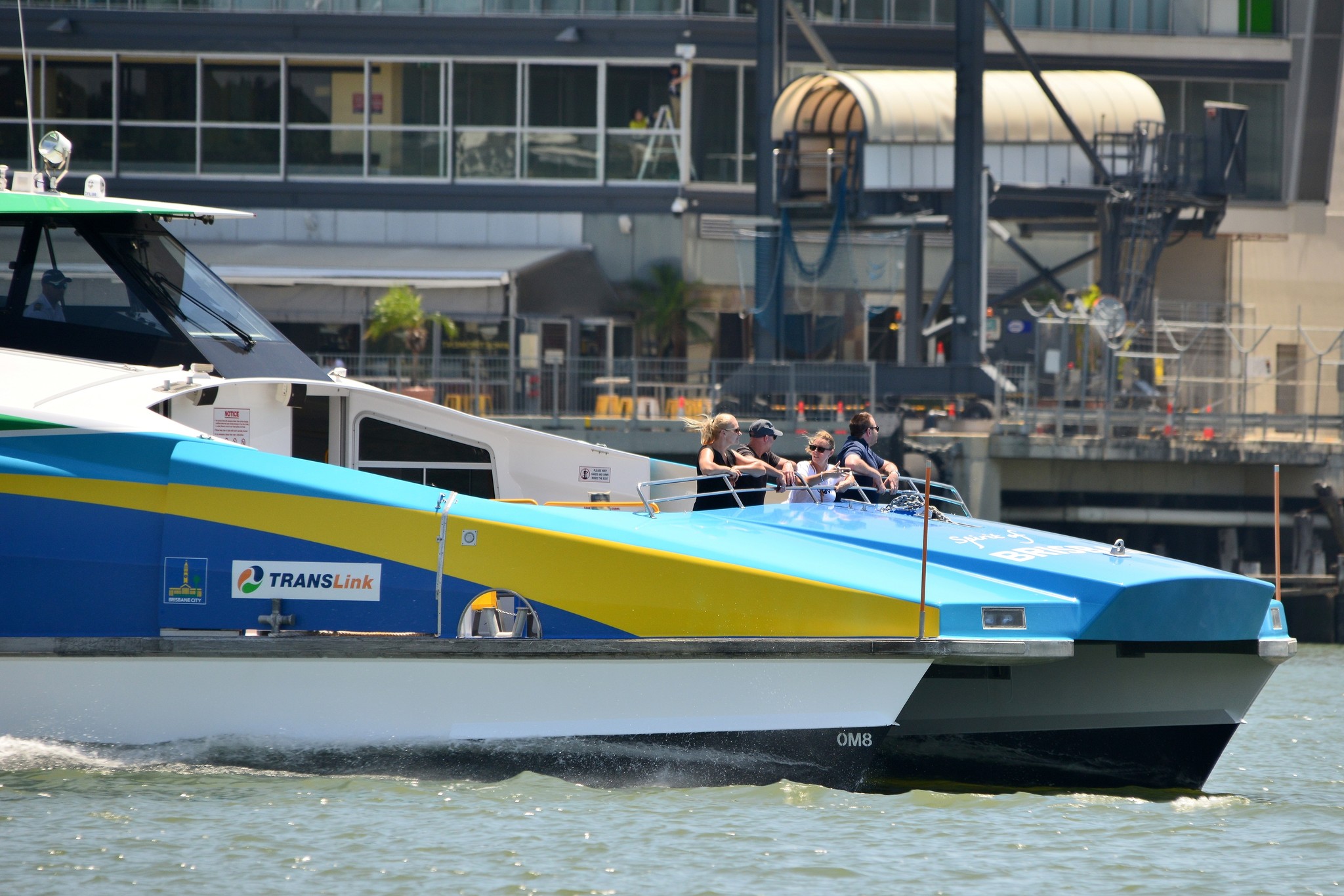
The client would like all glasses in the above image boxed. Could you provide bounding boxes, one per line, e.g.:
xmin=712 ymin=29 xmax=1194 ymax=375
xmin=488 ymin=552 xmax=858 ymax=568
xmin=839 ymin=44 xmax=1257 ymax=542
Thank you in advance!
xmin=719 ymin=426 xmax=740 ymax=434
xmin=769 ymin=434 xmax=777 ymax=440
xmin=863 ymin=426 xmax=879 ymax=433
xmin=810 ymin=445 xmax=832 ymax=453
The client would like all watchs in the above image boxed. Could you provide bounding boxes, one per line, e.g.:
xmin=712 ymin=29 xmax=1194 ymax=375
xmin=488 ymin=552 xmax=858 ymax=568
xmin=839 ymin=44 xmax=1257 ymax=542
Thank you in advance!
xmin=847 ymin=479 xmax=854 ymax=488
xmin=890 ymin=470 xmax=900 ymax=477
xmin=785 ymin=461 xmax=793 ymax=467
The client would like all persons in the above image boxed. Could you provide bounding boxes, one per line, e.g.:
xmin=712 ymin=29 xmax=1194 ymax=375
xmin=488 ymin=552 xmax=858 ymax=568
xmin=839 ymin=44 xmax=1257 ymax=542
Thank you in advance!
xmin=733 ymin=419 xmax=797 ymax=507
xmin=23 ymin=269 xmax=72 ymax=322
xmin=781 ymin=428 xmax=855 ymax=504
xmin=667 ymin=64 xmax=689 ymax=128
xmin=833 ymin=412 xmax=899 ymax=504
xmin=629 ymin=109 xmax=649 ymax=176
xmin=1301 ymin=481 xmax=1344 ymax=569
xmin=680 ymin=411 xmax=766 ymax=511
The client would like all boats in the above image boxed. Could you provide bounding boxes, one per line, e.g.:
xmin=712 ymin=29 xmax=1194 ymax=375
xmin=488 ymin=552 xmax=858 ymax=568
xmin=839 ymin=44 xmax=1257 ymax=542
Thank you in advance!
xmin=0 ymin=2 xmax=1298 ymax=795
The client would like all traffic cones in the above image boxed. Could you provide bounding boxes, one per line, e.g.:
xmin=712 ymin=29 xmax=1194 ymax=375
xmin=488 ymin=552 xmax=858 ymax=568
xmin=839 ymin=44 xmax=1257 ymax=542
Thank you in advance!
xmin=795 ymin=401 xmax=808 ymax=435
xmin=935 ymin=343 xmax=945 ymax=367
xmin=678 ymin=397 xmax=688 ymax=418
xmin=947 ymin=402 xmax=957 ymax=422
xmin=1202 ymin=405 xmax=1216 ymax=441
xmin=836 ymin=400 xmax=847 ymax=435
xmin=1164 ymin=405 xmax=1175 ymax=436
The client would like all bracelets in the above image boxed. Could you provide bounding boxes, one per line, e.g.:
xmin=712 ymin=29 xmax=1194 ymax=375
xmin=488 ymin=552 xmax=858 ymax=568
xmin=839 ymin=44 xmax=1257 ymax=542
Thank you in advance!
xmin=820 ymin=471 xmax=825 ymax=482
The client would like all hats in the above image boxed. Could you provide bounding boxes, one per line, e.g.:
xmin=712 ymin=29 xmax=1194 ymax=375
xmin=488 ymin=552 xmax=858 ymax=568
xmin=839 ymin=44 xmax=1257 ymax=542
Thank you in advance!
xmin=749 ymin=419 xmax=783 ymax=437
